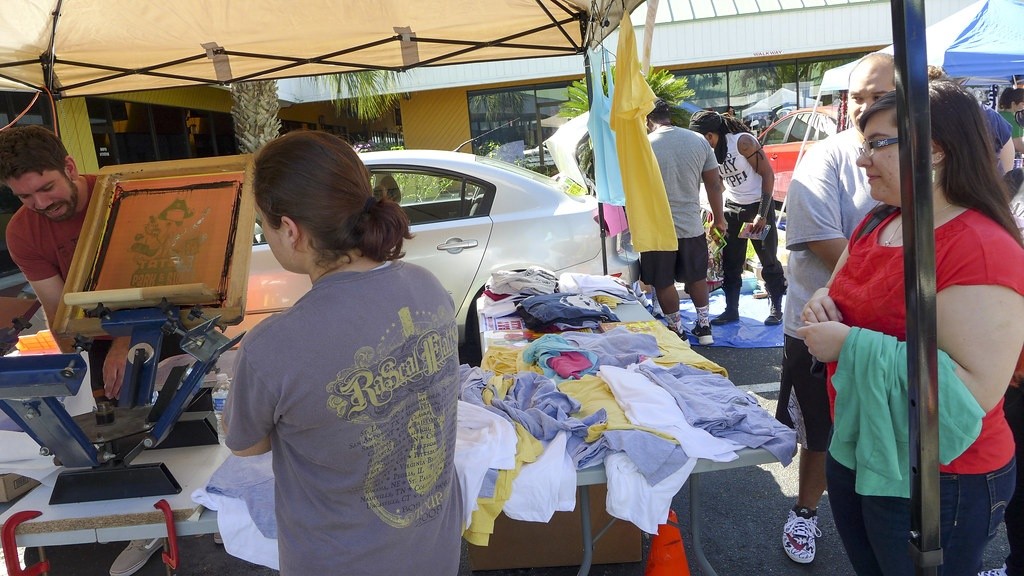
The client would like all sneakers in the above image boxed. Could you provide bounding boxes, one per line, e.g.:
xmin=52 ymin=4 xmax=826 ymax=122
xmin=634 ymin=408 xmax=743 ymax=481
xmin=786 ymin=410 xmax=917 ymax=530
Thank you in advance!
xmin=669 ymin=328 xmax=691 ymax=347
xmin=692 ymin=321 xmax=714 ymax=346
xmin=214 ymin=532 xmax=223 ymax=544
xmin=782 ymin=500 xmax=822 ymax=563
xmin=110 ymin=537 xmax=164 ymax=576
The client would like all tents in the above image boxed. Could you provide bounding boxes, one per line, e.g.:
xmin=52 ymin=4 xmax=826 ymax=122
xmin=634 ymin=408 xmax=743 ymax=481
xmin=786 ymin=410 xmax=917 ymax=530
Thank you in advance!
xmin=741 ymin=88 xmax=823 ymax=132
xmin=1 ymin=0 xmax=652 ymax=274
xmin=775 ymin=0 xmax=1024 ymax=232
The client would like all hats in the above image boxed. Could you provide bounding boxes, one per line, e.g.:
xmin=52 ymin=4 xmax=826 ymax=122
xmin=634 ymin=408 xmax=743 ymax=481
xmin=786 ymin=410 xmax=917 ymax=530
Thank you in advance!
xmin=651 ymin=98 xmax=669 ymax=112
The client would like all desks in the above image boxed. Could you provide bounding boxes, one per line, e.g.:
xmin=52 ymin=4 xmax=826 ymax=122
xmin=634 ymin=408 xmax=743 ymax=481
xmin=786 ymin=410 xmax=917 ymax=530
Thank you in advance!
xmin=0 ymin=271 xmax=796 ymax=575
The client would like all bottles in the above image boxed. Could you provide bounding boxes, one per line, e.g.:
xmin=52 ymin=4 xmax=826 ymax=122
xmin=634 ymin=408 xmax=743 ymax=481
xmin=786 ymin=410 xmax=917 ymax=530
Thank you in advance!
xmin=210 ymin=373 xmax=231 ymax=449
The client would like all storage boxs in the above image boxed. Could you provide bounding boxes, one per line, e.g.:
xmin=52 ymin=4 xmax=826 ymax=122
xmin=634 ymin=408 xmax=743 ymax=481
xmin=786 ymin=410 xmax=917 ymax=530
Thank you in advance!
xmin=469 ymin=481 xmax=642 ymax=570
xmin=1 ymin=470 xmax=38 ymax=504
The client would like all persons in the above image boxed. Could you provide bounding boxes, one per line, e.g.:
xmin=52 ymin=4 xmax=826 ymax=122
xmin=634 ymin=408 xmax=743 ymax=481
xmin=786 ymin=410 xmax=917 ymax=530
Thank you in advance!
xmin=688 ymin=110 xmax=785 ymax=325
xmin=220 ymin=129 xmax=463 ymax=576
xmin=998 ymin=87 xmax=1024 ymax=153
xmin=796 ymin=79 xmax=1024 ymax=576
xmin=647 ymin=95 xmax=726 ymax=346
xmin=0 ymin=125 xmax=214 ymax=411
xmin=726 ymin=106 xmax=734 ymax=115
xmin=928 ymin=66 xmax=1015 ymax=171
xmin=782 ymin=53 xmax=895 ymax=563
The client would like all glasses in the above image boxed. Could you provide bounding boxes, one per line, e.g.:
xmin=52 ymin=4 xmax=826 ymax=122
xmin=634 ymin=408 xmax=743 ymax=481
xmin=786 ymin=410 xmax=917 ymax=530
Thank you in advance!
xmin=858 ymin=137 xmax=898 ymax=157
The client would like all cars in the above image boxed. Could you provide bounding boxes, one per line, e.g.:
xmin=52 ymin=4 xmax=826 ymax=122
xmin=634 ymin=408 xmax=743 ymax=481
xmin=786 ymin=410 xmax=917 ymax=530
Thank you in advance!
xmin=214 ymin=107 xmax=639 ymax=355
xmin=756 ymin=103 xmax=855 ymax=207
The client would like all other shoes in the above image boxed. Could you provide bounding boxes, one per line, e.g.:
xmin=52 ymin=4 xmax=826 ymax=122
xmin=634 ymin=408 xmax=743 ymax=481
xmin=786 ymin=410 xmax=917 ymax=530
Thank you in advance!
xmin=977 ymin=562 xmax=1008 ymax=576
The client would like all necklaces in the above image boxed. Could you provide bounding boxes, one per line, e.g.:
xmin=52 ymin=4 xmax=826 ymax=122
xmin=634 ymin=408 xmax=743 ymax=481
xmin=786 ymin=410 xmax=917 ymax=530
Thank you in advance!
xmin=884 ymin=203 xmax=952 ymax=247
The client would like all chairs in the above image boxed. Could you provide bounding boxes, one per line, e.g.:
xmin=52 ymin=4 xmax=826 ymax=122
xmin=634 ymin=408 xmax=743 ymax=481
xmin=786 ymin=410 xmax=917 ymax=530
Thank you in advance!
xmin=379 ymin=176 xmax=403 ymax=206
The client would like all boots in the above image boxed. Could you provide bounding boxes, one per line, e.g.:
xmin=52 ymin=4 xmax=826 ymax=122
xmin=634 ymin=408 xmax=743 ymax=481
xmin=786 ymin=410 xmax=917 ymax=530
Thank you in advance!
xmin=711 ymin=286 xmax=741 ymax=325
xmin=765 ymin=287 xmax=782 ymax=325
